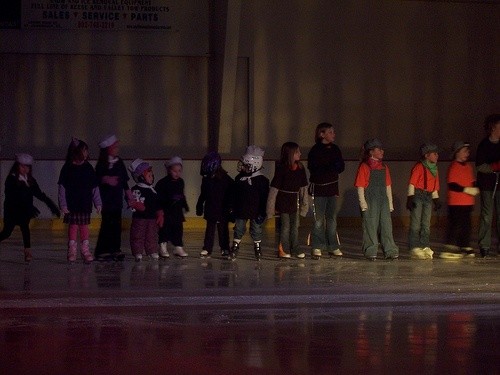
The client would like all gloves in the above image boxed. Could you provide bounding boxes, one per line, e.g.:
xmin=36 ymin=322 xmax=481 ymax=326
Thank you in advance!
xmin=406 ymin=196 xmax=416 ymax=211
xmin=433 ymin=198 xmax=442 ymax=211
xmin=196 ymin=204 xmax=203 ymax=217
xmin=256 ymin=212 xmax=266 ymax=224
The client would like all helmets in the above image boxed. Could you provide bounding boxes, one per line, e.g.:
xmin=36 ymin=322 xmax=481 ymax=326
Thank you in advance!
xmin=241 ymin=153 xmax=263 ymax=171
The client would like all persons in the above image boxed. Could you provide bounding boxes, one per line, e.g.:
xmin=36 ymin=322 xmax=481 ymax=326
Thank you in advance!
xmin=439 ymin=140 xmax=479 ymax=259
xmin=475 ymin=113 xmax=500 ymax=257
xmin=155 ymin=154 xmax=190 ymax=259
xmin=95 ymin=134 xmax=135 ymax=260
xmin=0 ymin=152 xmax=60 ymax=262
xmin=266 ymin=142 xmax=308 ymax=259
xmin=196 ymin=151 xmax=235 ymax=259
xmin=57 ymin=135 xmax=102 ymax=264
xmin=124 ymin=157 xmax=164 ymax=262
xmin=406 ymin=144 xmax=442 ymax=260
xmin=307 ymin=122 xmax=345 ymax=261
xmin=228 ymin=144 xmax=269 ymax=260
xmin=353 ymin=138 xmax=400 ymax=262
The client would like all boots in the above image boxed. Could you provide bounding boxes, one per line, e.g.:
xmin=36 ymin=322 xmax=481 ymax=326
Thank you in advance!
xmin=67 ymin=240 xmax=77 ymax=261
xmin=79 ymin=239 xmax=93 ymax=264
xmin=172 ymin=245 xmax=188 ymax=256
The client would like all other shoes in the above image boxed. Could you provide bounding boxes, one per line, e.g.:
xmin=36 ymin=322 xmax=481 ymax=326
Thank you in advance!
xmin=24 ymin=247 xmax=33 ymax=262
xmin=151 ymin=253 xmax=160 ymax=258
xmin=135 ymin=253 xmax=143 ymax=261
xmin=220 ymin=250 xmax=229 ymax=256
xmin=232 ymin=242 xmax=239 ymax=251
xmin=254 ymin=244 xmax=262 ymax=256
xmin=200 ymin=249 xmax=209 ymax=255
xmin=112 ymin=252 xmax=125 ymax=260
xmin=280 ymin=254 xmax=291 ymax=258
xmin=366 ymin=241 xmax=499 ymax=262
xmin=312 ymin=248 xmax=322 ymax=256
xmin=159 ymin=242 xmax=170 ymax=257
xmin=328 ymin=248 xmax=343 ymax=256
xmin=97 ymin=252 xmax=112 ymax=260
xmin=297 ymin=253 xmax=305 ymax=258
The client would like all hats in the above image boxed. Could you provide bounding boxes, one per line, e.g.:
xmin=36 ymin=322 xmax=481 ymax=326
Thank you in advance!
xmin=129 ymin=158 xmax=147 ymax=173
xmin=99 ymin=135 xmax=117 ymax=149
xmin=364 ymin=139 xmax=384 ymax=151
xmin=449 ymin=140 xmax=471 ymax=160
xmin=15 ymin=153 xmax=33 ymax=165
